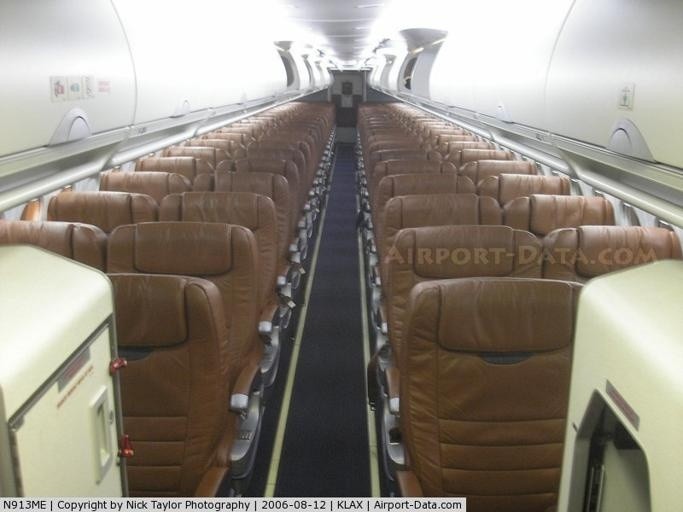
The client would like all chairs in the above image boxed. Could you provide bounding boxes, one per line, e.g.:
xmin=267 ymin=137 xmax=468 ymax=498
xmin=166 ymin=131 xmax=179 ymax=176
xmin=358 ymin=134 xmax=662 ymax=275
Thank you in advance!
xmin=0 ymin=95 xmax=683 ymax=512
